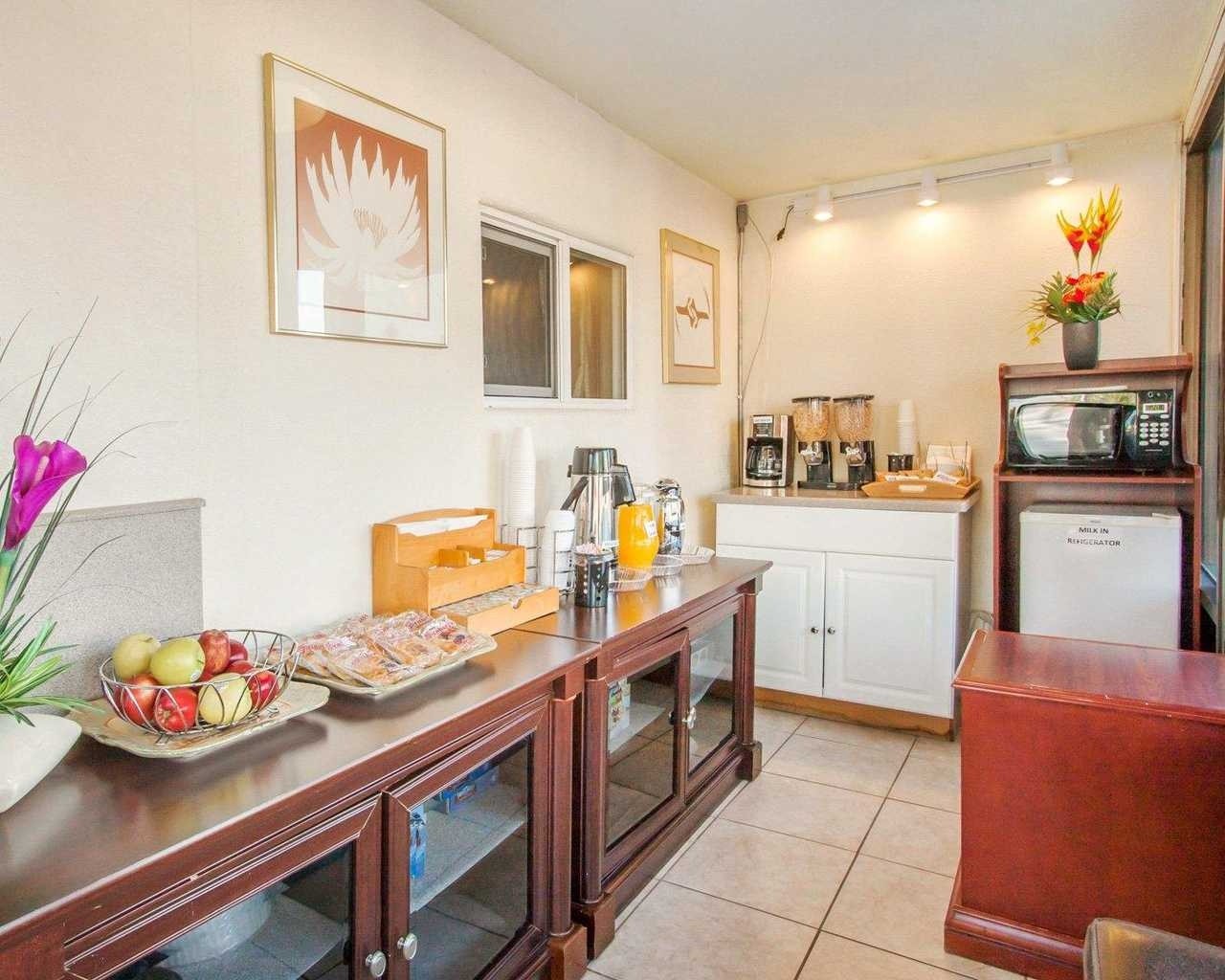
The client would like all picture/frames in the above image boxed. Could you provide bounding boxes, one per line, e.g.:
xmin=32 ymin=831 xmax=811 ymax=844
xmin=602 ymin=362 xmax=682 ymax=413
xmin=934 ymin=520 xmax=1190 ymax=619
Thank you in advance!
xmin=659 ymin=228 xmax=721 ymax=385
xmin=264 ymin=53 xmax=448 ymax=348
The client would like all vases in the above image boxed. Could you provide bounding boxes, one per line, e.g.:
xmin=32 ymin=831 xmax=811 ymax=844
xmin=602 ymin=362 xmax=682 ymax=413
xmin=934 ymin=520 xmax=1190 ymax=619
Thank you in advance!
xmin=0 ymin=712 xmax=82 ymax=813
xmin=1062 ymin=320 xmax=1098 ymax=370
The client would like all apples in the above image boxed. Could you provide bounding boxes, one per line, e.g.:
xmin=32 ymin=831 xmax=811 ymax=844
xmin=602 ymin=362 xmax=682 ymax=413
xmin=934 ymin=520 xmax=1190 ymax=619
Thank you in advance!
xmin=112 ymin=628 xmax=279 ymax=733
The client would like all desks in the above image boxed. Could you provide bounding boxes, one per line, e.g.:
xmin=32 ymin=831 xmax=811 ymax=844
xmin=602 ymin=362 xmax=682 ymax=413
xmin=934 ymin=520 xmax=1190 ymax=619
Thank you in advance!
xmin=945 ymin=628 xmax=1225 ymax=980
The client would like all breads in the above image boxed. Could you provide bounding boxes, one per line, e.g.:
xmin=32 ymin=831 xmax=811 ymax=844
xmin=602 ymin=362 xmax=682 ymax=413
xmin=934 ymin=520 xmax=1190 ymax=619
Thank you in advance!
xmin=266 ymin=614 xmax=474 ymax=686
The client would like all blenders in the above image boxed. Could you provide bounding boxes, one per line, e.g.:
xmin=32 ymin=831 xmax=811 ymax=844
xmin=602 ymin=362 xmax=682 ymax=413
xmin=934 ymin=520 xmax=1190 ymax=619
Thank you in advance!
xmin=791 ymin=396 xmax=838 ymax=489
xmin=833 ymin=393 xmax=874 ymax=490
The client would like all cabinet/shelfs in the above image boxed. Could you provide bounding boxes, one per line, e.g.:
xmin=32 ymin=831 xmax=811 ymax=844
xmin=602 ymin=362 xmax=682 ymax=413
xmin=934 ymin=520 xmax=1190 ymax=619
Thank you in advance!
xmin=371 ymin=508 xmax=560 ymax=637
xmin=711 ymin=487 xmax=984 ymax=741
xmin=993 ymin=352 xmax=1202 ymax=651
xmin=0 ymin=556 xmax=773 ymax=980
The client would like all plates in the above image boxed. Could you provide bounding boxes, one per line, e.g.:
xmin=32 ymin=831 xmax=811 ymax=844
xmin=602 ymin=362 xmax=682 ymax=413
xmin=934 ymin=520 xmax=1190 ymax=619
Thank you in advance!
xmin=64 ymin=678 xmax=330 ymax=758
xmin=673 ymin=545 xmax=716 ymax=565
xmin=609 ymin=566 xmax=653 ymax=592
xmin=651 ymin=555 xmax=685 ymax=577
xmin=248 ymin=631 xmax=497 ymax=697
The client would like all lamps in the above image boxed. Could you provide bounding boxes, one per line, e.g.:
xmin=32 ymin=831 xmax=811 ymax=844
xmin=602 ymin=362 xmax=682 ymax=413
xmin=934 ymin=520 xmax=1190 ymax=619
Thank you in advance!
xmin=813 ymin=184 xmax=834 ymax=221
xmin=917 ymin=174 xmax=940 ymax=206
xmin=1047 ymin=142 xmax=1073 ymax=186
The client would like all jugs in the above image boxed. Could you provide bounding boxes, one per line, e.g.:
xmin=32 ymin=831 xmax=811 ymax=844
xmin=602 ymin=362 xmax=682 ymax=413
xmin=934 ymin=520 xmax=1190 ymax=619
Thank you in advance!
xmin=559 ymin=447 xmax=636 ymax=553
xmin=618 ymin=483 xmax=665 ymax=566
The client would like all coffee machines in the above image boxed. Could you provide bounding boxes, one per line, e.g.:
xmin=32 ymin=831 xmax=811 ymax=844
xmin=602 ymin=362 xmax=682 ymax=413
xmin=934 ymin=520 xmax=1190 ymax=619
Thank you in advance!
xmin=742 ymin=414 xmax=795 ymax=487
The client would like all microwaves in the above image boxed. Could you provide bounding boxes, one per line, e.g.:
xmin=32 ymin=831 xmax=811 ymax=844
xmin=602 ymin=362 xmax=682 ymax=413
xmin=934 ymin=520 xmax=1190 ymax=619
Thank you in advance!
xmin=1006 ymin=386 xmax=1185 ymax=471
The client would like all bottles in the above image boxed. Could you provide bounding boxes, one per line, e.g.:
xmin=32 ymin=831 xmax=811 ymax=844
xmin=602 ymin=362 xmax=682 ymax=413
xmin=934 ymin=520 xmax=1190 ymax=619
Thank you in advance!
xmin=655 ymin=477 xmax=685 ymax=556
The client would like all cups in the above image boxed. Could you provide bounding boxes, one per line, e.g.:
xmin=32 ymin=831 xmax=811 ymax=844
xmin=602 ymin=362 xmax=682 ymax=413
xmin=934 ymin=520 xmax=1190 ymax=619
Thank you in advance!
xmin=888 ymin=455 xmax=913 ymax=472
xmin=898 ymin=401 xmax=919 ymax=458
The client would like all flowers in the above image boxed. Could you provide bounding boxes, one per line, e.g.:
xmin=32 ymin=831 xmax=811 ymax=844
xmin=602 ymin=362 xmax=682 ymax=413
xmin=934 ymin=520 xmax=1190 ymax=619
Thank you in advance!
xmin=0 ymin=297 xmax=177 ymax=727
xmin=1019 ymin=184 xmax=1124 ymax=346
